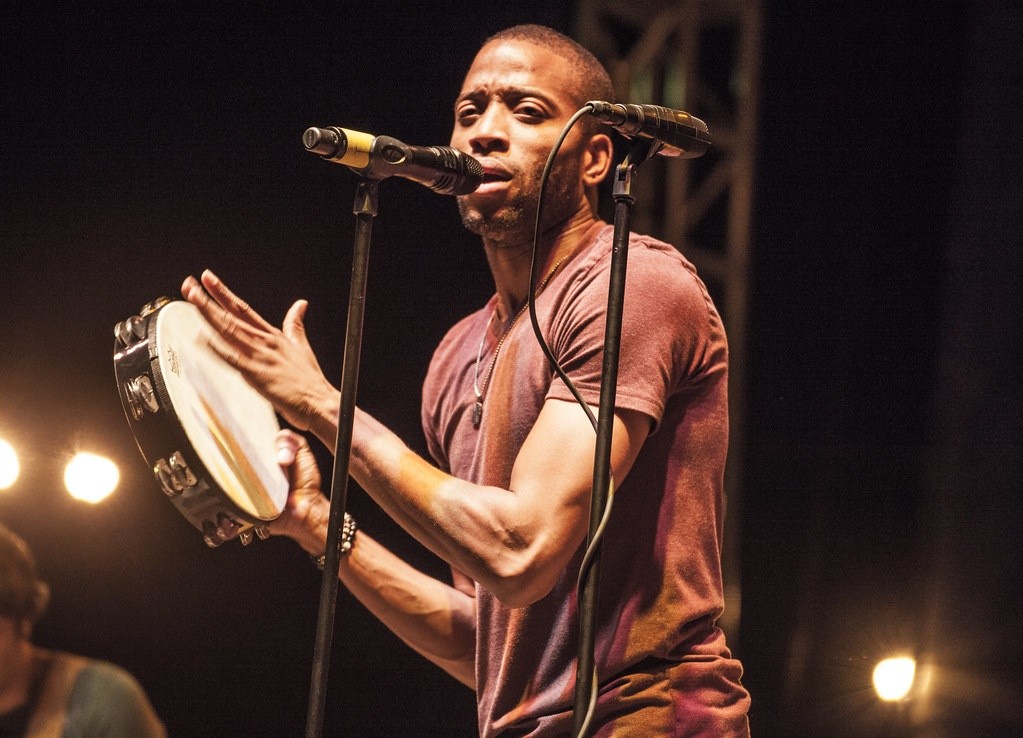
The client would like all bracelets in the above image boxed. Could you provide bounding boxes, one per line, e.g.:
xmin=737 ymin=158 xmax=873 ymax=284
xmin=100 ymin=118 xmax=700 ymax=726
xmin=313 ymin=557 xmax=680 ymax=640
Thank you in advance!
xmin=314 ymin=512 xmax=356 ymax=572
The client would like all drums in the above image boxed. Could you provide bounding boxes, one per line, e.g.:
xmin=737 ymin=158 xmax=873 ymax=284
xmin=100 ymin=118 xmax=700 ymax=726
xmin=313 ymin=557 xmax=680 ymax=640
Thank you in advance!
xmin=112 ymin=299 xmax=288 ymax=543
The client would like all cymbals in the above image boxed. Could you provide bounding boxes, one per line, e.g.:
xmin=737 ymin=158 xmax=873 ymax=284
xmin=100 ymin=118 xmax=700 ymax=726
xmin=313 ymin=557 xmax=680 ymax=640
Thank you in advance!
xmin=109 ymin=295 xmax=292 ymax=550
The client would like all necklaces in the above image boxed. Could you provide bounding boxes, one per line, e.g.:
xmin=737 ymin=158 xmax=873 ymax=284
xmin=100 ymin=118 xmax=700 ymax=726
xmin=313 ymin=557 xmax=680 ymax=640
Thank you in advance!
xmin=472 ymin=255 xmax=569 ymax=425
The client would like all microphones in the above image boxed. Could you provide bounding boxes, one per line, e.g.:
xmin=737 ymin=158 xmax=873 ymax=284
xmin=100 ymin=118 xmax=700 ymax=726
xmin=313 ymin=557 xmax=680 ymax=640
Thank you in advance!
xmin=586 ymin=100 xmax=712 ymax=158
xmin=304 ymin=125 xmax=484 ymax=195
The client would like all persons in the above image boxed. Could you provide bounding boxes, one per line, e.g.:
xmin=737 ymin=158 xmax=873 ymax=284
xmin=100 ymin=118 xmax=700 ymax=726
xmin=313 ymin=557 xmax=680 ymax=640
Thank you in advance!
xmin=181 ymin=26 xmax=752 ymax=738
xmin=0 ymin=525 xmax=163 ymax=738
